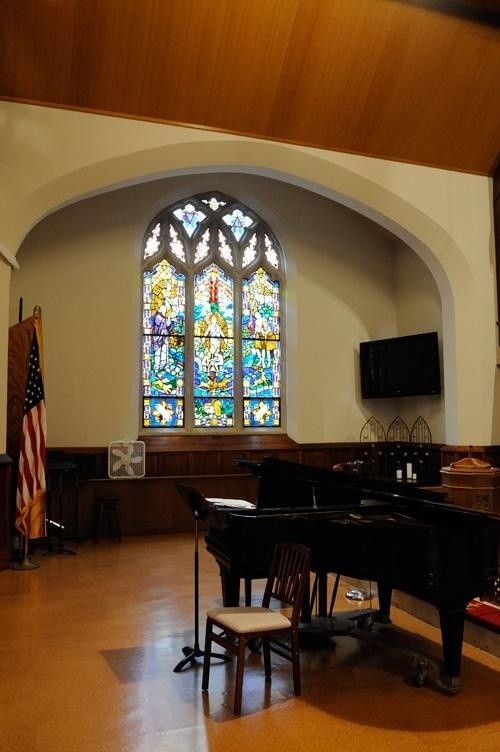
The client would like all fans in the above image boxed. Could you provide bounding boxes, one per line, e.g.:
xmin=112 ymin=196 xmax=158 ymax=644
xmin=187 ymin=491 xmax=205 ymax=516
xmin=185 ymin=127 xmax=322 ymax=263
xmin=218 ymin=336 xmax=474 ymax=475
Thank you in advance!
xmin=107 ymin=440 xmax=148 ymax=480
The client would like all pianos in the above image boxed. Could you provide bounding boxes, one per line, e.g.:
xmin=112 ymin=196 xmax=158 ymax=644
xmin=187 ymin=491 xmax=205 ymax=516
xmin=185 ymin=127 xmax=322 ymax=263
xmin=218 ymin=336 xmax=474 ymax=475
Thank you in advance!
xmin=205 ymin=455 xmax=500 ymax=697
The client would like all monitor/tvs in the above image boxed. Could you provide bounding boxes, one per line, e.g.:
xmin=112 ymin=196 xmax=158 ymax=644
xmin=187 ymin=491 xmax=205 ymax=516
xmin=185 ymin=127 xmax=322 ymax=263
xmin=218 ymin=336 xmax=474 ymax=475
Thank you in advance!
xmin=359 ymin=331 xmax=441 ymax=399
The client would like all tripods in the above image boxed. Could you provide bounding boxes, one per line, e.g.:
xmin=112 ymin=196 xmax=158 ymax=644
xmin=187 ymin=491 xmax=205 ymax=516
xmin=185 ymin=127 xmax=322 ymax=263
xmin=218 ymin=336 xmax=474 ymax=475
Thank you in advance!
xmin=174 ymin=516 xmax=232 ymax=671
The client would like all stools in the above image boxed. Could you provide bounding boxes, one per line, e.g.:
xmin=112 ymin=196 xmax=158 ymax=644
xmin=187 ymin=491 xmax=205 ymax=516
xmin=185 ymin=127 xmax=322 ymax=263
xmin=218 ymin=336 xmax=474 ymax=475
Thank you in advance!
xmin=92 ymin=494 xmax=121 ymax=544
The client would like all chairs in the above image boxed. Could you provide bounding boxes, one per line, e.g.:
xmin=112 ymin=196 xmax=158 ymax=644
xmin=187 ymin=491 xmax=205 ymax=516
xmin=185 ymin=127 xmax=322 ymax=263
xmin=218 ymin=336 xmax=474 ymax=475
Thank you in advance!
xmin=201 ymin=535 xmax=311 ymax=717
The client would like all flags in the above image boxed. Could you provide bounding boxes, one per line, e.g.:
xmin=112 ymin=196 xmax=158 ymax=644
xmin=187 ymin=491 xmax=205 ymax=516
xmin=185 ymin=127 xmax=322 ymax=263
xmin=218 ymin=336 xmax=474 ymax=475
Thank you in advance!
xmin=11 ymin=327 xmax=50 ymax=546
